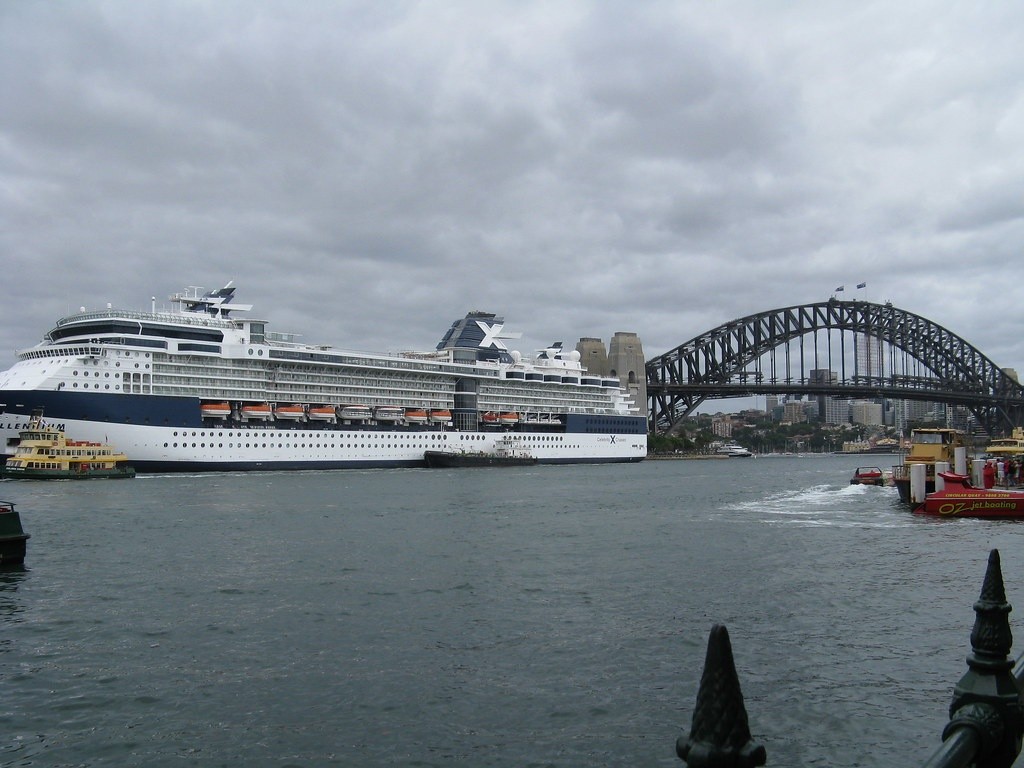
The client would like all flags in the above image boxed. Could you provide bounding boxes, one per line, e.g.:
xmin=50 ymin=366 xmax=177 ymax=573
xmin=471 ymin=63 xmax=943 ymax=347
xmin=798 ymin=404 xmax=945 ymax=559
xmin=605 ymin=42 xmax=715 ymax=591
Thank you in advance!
xmin=857 ymin=282 xmax=867 ymax=289
xmin=836 ymin=286 xmax=845 ymax=291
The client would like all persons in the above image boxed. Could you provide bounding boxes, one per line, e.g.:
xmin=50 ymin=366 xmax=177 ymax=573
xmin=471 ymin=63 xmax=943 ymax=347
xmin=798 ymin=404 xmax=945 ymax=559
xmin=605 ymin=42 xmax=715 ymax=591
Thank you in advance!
xmin=1006 ymin=459 xmax=1016 ymax=486
xmin=993 ymin=458 xmax=999 ymax=478
xmin=984 ymin=462 xmax=994 ymax=489
xmin=1005 ymin=458 xmax=1024 ymax=483
xmin=967 ymin=459 xmax=974 ymax=486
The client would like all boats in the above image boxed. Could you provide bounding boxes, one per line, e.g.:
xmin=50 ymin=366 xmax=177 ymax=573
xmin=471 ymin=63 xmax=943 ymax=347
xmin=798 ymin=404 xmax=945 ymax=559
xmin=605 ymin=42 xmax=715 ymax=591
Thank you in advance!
xmin=306 ymin=406 xmax=336 ymax=421
xmin=240 ymin=404 xmax=270 ymax=419
xmin=981 ymin=425 xmax=1024 ymax=463
xmin=482 ymin=410 xmax=500 ymax=425
xmin=199 ymin=400 xmax=232 ymax=420
xmin=890 ymin=419 xmax=977 ymax=508
xmin=713 ymin=442 xmax=752 ymax=456
xmin=1 ymin=400 xmax=137 ymax=479
xmin=850 ymin=466 xmax=884 ymax=487
xmin=0 ymin=501 xmax=31 ymax=567
xmin=498 ymin=411 xmax=518 ymax=424
xmin=426 ymin=410 xmax=452 ymax=422
xmin=372 ymin=406 xmax=404 ymax=422
xmin=273 ymin=403 xmax=304 ymax=420
xmin=405 ymin=409 xmax=427 ymax=423
xmin=336 ymin=404 xmax=373 ymax=421
xmin=423 ymin=428 xmax=538 ymax=469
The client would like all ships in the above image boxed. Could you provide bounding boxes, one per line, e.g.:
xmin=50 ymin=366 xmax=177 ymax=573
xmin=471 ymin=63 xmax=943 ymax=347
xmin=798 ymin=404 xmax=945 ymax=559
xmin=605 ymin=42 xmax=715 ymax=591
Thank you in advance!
xmin=0 ymin=275 xmax=648 ymax=477
